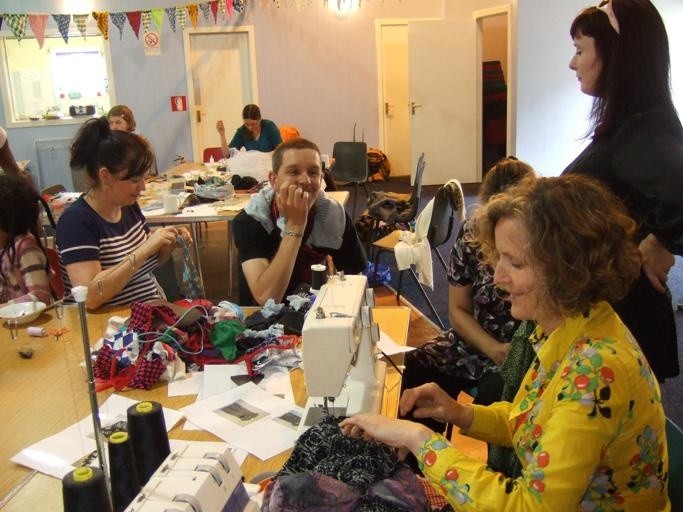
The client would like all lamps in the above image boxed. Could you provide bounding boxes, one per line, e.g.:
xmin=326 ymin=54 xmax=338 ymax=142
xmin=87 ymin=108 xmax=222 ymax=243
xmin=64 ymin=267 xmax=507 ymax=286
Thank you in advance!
xmin=328 ymin=0 xmax=360 ymax=22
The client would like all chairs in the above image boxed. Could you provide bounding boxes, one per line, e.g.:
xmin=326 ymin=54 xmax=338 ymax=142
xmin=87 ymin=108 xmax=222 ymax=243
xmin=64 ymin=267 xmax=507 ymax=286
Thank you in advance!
xmin=203 ymin=147 xmax=224 ymax=163
xmin=364 ymin=152 xmax=425 ymax=252
xmin=366 ymin=161 xmax=426 ymax=263
xmin=329 ymin=142 xmax=372 ymax=220
xmin=373 ymin=186 xmax=454 ymax=331
xmin=40 ymin=184 xmax=66 ymax=196
xmin=278 ymin=128 xmax=299 ymax=142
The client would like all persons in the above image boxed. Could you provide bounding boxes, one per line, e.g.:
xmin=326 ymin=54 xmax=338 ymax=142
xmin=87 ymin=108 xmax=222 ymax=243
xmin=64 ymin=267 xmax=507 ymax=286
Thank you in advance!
xmin=217 ymin=102 xmax=281 ymax=156
xmin=0 ymin=173 xmax=52 ymax=306
xmin=0 ymin=129 xmax=30 ymax=176
xmin=51 ymin=117 xmax=193 ymax=312
xmin=554 ymin=0 xmax=683 ymax=380
xmin=335 ymin=176 xmax=670 ymax=512
xmin=108 ymin=105 xmax=136 ymax=134
xmin=231 ymin=138 xmax=367 ymax=307
xmin=399 ymin=155 xmax=535 ymax=432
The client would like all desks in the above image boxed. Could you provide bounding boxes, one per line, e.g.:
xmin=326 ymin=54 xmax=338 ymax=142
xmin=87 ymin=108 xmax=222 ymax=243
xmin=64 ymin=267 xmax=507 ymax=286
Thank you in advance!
xmin=1 ymin=306 xmax=412 ymax=512
xmin=40 ymin=192 xmax=350 ymax=297
xmin=145 ymin=162 xmax=264 ymax=193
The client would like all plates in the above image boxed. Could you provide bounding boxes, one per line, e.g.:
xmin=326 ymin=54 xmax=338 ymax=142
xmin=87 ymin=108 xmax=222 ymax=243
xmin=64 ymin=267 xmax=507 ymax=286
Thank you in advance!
xmin=0 ymin=303 xmax=46 ymax=323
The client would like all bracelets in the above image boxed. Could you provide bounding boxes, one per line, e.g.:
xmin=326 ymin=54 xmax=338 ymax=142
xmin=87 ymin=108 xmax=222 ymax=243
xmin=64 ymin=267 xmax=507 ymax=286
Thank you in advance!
xmin=284 ymin=230 xmax=303 ymax=238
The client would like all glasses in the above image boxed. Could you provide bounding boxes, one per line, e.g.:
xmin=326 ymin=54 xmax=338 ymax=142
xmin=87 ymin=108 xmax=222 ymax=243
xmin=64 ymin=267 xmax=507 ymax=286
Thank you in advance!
xmin=596 ymin=0 xmax=619 ymax=34
